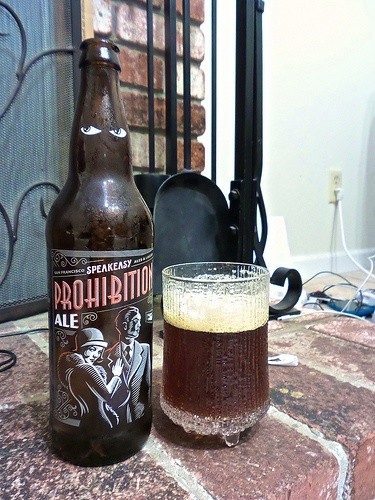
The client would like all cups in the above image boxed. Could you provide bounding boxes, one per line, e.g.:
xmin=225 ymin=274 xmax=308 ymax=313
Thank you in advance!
xmin=162 ymin=262 xmax=269 ymax=447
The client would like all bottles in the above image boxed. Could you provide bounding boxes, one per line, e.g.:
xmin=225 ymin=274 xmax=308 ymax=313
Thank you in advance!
xmin=46 ymin=37 xmax=153 ymax=468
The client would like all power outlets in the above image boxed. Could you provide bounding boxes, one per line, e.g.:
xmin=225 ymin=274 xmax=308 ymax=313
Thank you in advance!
xmin=329 ymin=168 xmax=343 ymax=203
